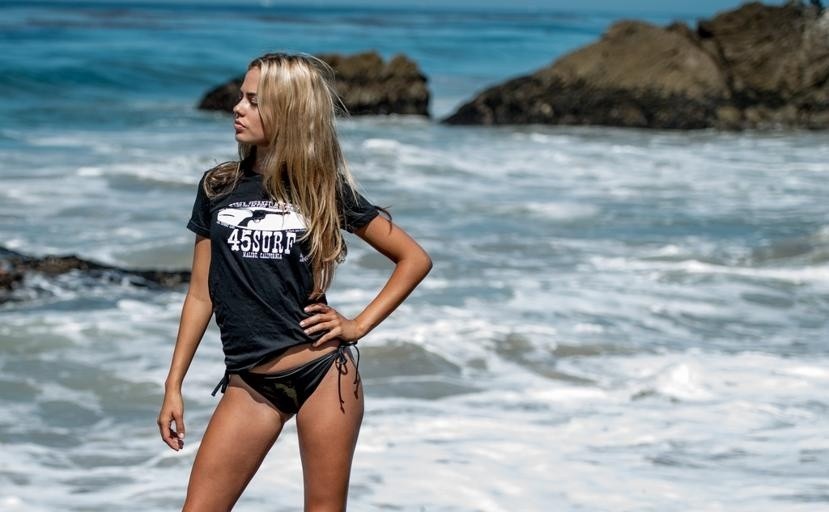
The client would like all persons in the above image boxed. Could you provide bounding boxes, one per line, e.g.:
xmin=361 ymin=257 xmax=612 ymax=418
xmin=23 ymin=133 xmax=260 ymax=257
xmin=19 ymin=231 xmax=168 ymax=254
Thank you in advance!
xmin=157 ymin=50 xmax=433 ymax=512
